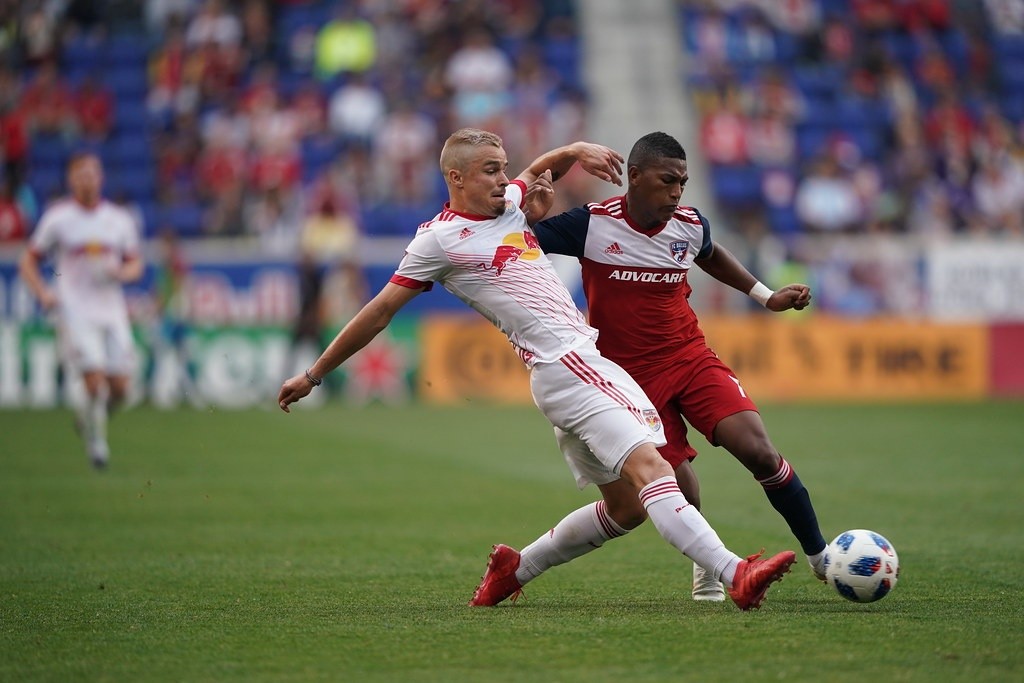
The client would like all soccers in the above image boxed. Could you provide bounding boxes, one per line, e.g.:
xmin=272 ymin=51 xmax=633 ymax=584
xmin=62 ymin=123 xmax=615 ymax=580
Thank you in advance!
xmin=824 ymin=529 xmax=901 ymax=605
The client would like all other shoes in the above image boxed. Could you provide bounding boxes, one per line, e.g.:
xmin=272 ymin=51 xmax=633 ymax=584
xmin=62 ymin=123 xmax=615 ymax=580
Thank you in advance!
xmin=72 ymin=406 xmax=109 ymax=466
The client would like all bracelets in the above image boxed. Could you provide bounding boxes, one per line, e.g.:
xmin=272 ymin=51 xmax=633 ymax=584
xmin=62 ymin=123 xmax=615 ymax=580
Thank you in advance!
xmin=304 ymin=367 xmax=324 ymax=387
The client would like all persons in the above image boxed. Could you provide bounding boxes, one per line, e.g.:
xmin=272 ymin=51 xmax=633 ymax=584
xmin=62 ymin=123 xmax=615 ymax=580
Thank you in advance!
xmin=519 ymin=130 xmax=832 ymax=604
xmin=0 ymin=0 xmax=1023 ymax=241
xmin=276 ymin=127 xmax=796 ymax=608
xmin=19 ymin=150 xmax=145 ymax=469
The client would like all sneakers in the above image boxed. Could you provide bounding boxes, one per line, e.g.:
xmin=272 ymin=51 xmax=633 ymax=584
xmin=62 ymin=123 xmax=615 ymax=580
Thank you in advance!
xmin=468 ymin=544 xmax=528 ymax=606
xmin=726 ymin=547 xmax=797 ymax=611
xmin=814 ymin=570 xmax=827 ymax=584
xmin=692 ymin=558 xmax=724 ymax=601
